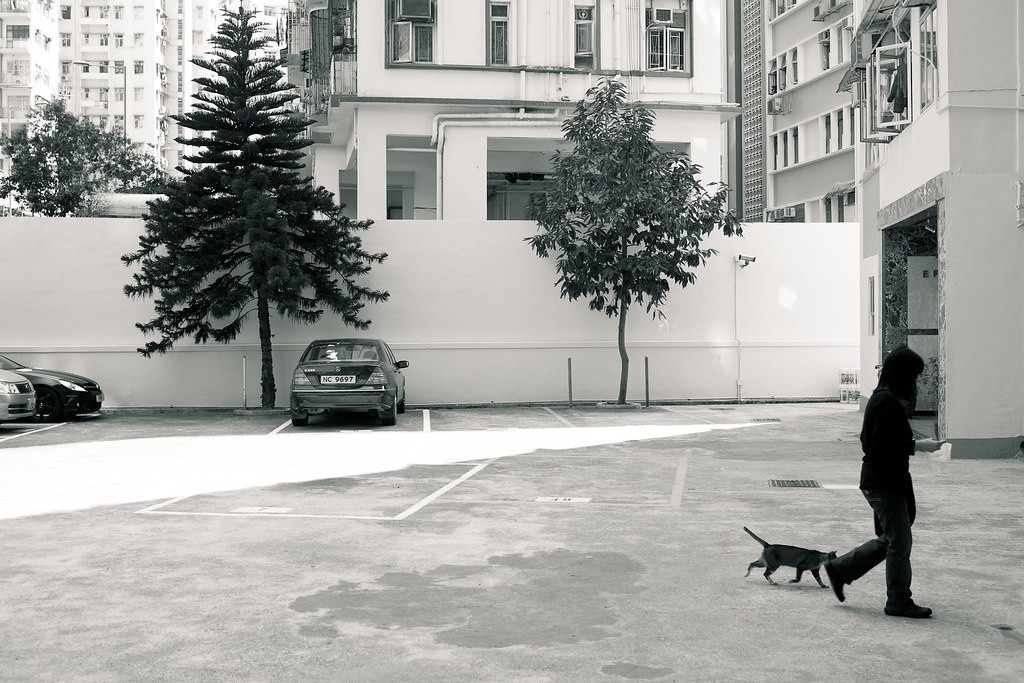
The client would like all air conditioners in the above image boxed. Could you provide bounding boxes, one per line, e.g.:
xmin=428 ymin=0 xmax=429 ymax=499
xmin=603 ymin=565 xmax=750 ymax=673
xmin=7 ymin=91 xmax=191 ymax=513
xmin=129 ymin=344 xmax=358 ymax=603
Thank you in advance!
xmin=767 ymin=98 xmax=782 ymax=113
xmin=651 ymin=7 xmax=673 ymax=24
xmin=769 ymin=208 xmax=796 ymax=220
xmin=842 ymin=17 xmax=852 ymax=30
xmin=814 ymin=1 xmax=829 ymax=19
xmin=851 ymin=82 xmax=867 ymax=108
xmin=817 ymin=31 xmax=830 ymax=44
xmin=829 ymin=0 xmax=846 ymax=12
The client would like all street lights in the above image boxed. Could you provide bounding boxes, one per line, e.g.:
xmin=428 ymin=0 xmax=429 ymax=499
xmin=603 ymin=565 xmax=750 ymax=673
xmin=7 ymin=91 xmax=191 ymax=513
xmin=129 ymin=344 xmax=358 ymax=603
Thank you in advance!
xmin=72 ymin=60 xmax=127 ymax=150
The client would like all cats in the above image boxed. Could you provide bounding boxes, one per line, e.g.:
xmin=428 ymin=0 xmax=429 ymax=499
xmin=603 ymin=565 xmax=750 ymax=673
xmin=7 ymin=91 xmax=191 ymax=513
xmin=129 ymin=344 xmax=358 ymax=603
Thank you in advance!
xmin=743 ymin=526 xmax=837 ymax=589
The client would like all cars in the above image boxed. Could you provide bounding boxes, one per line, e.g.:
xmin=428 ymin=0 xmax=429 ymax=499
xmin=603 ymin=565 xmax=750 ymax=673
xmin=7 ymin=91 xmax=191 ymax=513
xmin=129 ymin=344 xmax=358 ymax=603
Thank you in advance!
xmin=290 ymin=338 xmax=409 ymax=427
xmin=0 ymin=355 xmax=104 ymax=423
xmin=0 ymin=369 xmax=38 ymax=421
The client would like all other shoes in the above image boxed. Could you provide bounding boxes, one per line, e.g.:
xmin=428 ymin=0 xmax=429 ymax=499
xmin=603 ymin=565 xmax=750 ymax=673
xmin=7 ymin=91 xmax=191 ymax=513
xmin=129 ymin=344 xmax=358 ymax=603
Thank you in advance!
xmin=884 ymin=605 xmax=932 ymax=617
xmin=824 ymin=560 xmax=845 ymax=602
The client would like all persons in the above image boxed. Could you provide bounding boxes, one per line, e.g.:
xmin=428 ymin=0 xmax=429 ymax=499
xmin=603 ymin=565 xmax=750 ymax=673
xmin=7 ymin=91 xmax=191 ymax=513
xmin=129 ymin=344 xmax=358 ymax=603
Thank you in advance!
xmin=823 ymin=342 xmax=947 ymax=618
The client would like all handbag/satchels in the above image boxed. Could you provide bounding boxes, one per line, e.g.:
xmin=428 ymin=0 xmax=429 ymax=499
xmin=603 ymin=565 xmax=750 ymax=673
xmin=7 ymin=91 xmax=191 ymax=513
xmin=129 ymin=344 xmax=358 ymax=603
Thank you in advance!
xmin=874 ymin=472 xmax=917 ymax=538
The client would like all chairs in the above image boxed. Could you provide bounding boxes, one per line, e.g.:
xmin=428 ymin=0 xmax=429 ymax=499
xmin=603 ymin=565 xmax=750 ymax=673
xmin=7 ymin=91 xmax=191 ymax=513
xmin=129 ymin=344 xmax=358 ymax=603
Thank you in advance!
xmin=363 ymin=351 xmax=377 ymax=359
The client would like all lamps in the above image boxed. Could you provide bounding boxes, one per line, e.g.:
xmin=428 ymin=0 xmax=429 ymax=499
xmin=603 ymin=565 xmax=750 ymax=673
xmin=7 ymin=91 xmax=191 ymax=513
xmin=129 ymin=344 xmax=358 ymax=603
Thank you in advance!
xmin=925 ymin=218 xmax=935 ymax=233
